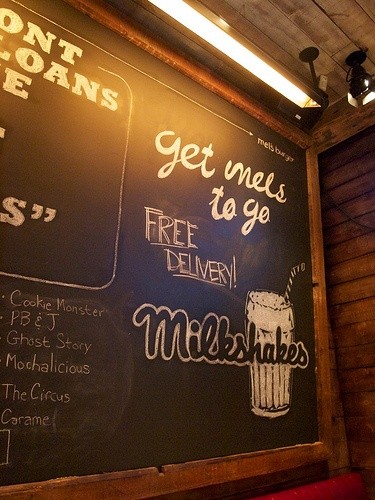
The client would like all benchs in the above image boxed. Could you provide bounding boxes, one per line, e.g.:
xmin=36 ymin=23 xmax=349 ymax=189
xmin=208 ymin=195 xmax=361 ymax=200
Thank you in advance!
xmin=248 ymin=473 xmax=372 ymax=500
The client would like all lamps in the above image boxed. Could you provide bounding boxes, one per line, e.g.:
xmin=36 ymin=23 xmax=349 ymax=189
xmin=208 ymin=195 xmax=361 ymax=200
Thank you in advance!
xmin=344 ymin=47 xmax=375 ymax=98
xmin=147 ymin=0 xmax=330 ymax=113
